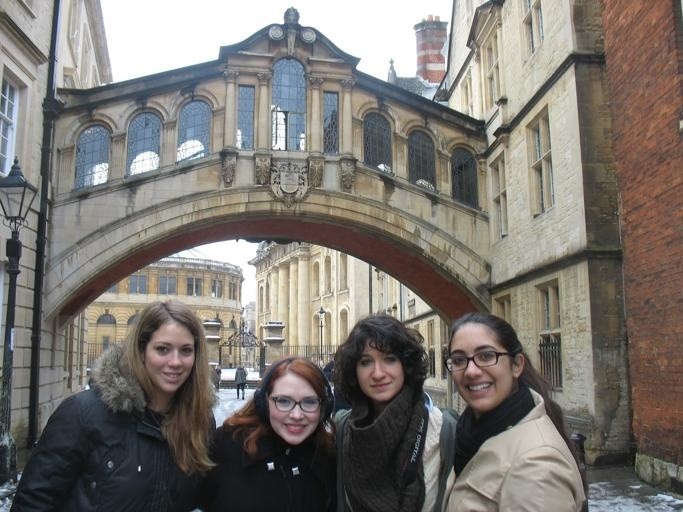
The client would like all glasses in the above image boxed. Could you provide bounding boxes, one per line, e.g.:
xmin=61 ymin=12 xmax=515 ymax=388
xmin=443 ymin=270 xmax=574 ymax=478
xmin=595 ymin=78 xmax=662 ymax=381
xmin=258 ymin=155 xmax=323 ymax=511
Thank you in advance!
xmin=445 ymin=350 xmax=516 ymax=373
xmin=272 ymin=396 xmax=325 ymax=413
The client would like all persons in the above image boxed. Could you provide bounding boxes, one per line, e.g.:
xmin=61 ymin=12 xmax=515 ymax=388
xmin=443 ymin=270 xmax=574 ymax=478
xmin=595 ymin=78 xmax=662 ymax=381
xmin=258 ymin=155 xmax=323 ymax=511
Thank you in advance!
xmin=328 ymin=313 xmax=460 ymax=512
xmin=213 ymin=368 xmax=221 ymax=389
xmin=441 ymin=311 xmax=586 ymax=512
xmin=9 ymin=301 xmax=219 ymax=512
xmin=235 ymin=364 xmax=247 ymax=400
xmin=215 ymin=355 xmax=338 ymax=511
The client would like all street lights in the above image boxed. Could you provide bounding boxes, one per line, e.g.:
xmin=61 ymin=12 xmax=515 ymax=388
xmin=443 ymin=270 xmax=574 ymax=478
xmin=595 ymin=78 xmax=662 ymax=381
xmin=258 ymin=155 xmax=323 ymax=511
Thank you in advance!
xmin=317 ymin=306 xmax=326 ymax=364
xmin=1 ymin=156 xmax=39 ymax=487
